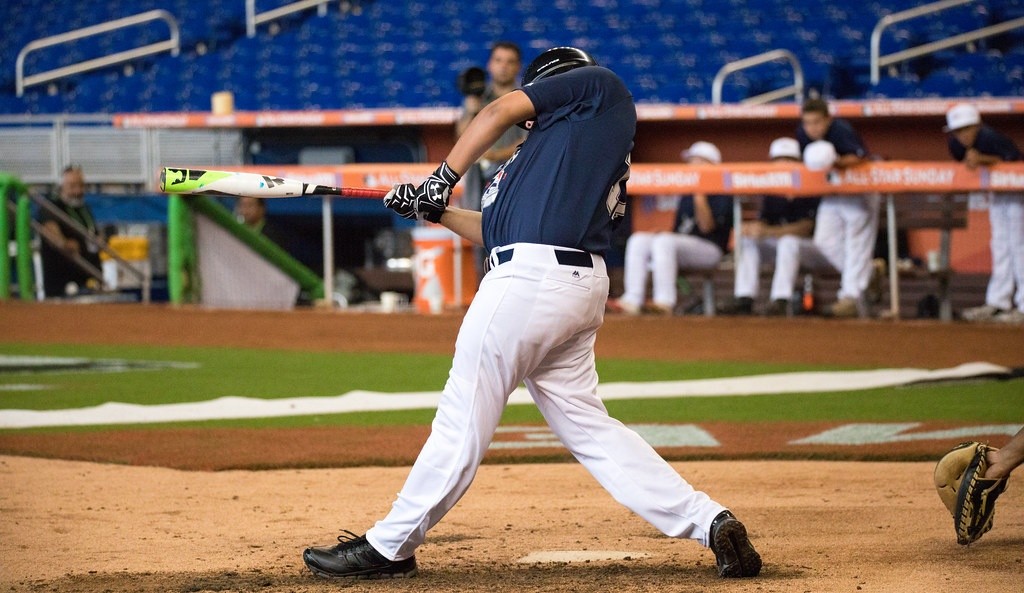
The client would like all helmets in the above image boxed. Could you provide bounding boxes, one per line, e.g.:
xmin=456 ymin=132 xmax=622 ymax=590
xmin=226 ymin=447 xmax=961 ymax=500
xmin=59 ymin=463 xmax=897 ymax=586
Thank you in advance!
xmin=516 ymin=46 xmax=597 ymax=130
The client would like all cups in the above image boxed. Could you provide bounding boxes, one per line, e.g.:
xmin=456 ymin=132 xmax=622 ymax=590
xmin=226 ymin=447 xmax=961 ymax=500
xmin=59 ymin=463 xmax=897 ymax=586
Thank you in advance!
xmin=926 ymin=252 xmax=940 ymax=272
xmin=210 ymin=92 xmax=234 ymax=116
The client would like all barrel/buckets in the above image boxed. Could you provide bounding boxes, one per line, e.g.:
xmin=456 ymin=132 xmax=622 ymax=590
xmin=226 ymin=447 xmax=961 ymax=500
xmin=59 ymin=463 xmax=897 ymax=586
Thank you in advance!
xmin=407 ymin=225 xmax=480 ymax=310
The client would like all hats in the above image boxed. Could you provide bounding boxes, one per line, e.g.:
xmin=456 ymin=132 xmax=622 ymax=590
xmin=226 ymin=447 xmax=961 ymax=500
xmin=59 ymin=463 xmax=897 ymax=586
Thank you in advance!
xmin=769 ymin=137 xmax=801 ymax=161
xmin=941 ymin=104 xmax=980 ymax=133
xmin=803 ymin=139 xmax=836 ymax=171
xmin=680 ymin=142 xmax=721 ymax=166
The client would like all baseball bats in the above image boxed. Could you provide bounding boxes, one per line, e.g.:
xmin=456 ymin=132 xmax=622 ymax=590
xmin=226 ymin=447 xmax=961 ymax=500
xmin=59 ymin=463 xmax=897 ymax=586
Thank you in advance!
xmin=156 ymin=165 xmax=390 ymax=199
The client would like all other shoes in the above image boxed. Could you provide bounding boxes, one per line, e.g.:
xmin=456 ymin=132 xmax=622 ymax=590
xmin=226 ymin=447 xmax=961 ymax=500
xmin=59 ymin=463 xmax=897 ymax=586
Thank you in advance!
xmin=639 ymin=303 xmax=671 ymax=317
xmin=603 ymin=295 xmax=623 ymax=313
xmin=965 ymin=305 xmax=1023 ymax=324
xmin=861 ymin=283 xmax=882 ymax=305
xmin=736 ymin=296 xmax=752 ymax=314
xmin=832 ymin=302 xmax=857 ymax=320
xmin=773 ymin=297 xmax=789 ymax=316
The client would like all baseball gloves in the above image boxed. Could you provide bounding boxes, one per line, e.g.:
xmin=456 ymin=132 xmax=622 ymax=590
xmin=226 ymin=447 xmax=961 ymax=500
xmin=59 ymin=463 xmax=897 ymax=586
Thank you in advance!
xmin=933 ymin=441 xmax=1009 ymax=547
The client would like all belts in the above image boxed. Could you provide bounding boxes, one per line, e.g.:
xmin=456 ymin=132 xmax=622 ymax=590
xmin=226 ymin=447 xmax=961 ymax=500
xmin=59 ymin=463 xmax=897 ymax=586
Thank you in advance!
xmin=485 ymin=247 xmax=593 ymax=272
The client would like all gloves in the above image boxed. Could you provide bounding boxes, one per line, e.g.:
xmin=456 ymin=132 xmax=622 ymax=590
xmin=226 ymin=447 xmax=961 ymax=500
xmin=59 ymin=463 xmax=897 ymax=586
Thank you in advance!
xmin=384 ymin=184 xmax=419 ymax=221
xmin=415 ymin=161 xmax=460 ymax=224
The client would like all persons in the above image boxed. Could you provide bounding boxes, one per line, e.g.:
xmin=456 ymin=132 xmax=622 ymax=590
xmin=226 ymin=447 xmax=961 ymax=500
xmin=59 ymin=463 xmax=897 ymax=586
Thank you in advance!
xmin=0 ymin=162 xmax=316 ymax=297
xmin=303 ymin=46 xmax=764 ymax=579
xmin=454 ymin=40 xmax=1024 ymax=322
xmin=933 ymin=429 xmax=1024 ymax=545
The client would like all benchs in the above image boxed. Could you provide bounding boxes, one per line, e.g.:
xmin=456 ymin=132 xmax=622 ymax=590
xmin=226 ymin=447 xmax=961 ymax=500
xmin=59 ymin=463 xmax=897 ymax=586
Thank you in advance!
xmin=671 ymin=185 xmax=967 ymax=324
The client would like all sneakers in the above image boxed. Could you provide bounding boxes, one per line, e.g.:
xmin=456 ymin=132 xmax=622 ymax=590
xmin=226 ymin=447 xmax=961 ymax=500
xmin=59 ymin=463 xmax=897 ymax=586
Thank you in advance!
xmin=302 ymin=529 xmax=418 ymax=580
xmin=709 ymin=509 xmax=762 ymax=579
